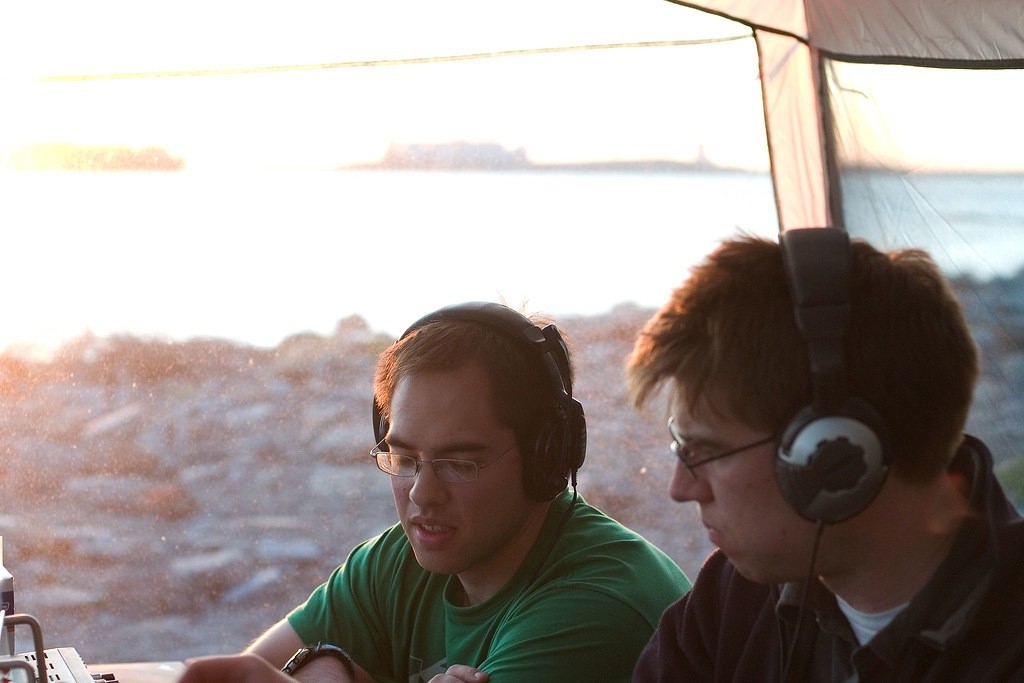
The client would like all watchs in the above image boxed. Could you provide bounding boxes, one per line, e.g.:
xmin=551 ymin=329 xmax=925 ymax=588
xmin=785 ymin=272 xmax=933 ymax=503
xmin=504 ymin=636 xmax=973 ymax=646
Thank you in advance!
xmin=281 ymin=641 xmax=356 ymax=681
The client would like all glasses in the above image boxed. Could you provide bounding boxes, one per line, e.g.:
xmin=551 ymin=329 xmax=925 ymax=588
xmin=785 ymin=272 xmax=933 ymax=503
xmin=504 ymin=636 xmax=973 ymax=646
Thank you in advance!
xmin=369 ymin=437 xmax=519 ymax=483
xmin=667 ymin=416 xmax=778 ymax=468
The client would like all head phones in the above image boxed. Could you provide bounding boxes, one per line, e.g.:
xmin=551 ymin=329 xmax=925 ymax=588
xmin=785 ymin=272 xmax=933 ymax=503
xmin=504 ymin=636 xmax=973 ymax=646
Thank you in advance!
xmin=372 ymin=302 xmax=588 ymax=503
xmin=778 ymin=227 xmax=891 ymax=527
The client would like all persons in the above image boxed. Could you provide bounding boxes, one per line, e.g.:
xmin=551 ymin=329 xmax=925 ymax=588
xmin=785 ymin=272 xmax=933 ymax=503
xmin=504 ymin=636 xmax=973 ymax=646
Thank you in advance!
xmin=178 ymin=236 xmax=1024 ymax=683
xmin=242 ymin=320 xmax=692 ymax=683
